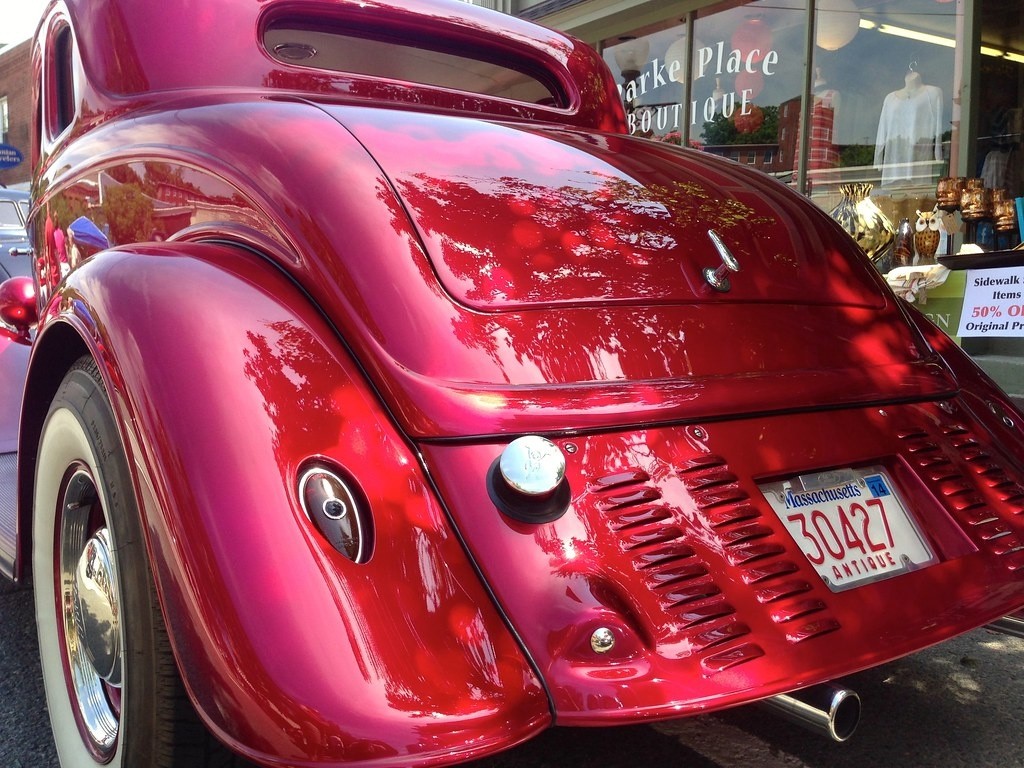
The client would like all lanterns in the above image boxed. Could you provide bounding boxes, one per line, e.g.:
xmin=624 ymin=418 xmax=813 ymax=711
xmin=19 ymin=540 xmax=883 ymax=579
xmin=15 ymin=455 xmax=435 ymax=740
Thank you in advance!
xmin=665 ymin=0 xmax=861 ymax=132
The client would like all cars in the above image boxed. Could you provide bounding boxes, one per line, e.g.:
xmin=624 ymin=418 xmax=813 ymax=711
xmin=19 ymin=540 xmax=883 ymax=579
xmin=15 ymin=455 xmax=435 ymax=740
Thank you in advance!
xmin=0 ymin=1 xmax=1024 ymax=768
xmin=0 ymin=188 xmax=34 ymax=285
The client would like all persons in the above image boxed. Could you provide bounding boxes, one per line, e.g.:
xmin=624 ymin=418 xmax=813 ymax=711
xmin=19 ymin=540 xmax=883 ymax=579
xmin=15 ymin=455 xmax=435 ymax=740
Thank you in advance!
xmin=634 ymin=86 xmax=738 ymax=151
xmin=794 ymin=79 xmax=842 ymax=188
xmin=872 ymin=71 xmax=945 ymax=183
